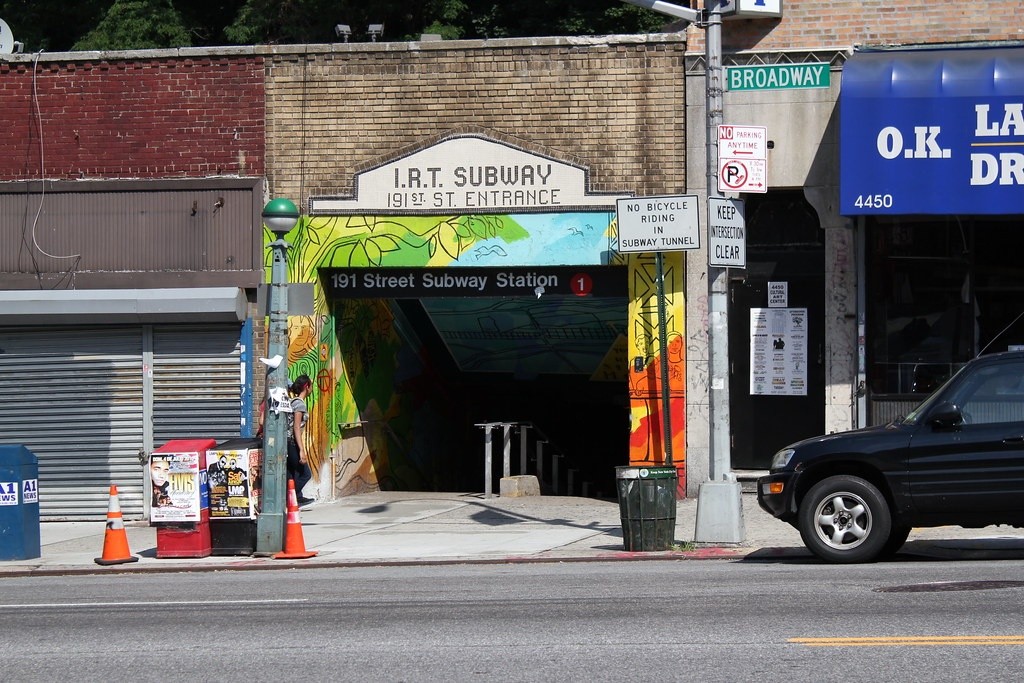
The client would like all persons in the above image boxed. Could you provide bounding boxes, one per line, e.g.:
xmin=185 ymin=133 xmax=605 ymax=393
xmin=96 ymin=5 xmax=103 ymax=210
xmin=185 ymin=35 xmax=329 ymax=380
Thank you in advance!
xmin=287 ymin=375 xmax=314 ymax=506
xmin=228 ymin=459 xmax=248 ymax=497
xmin=256 ymin=377 xmax=292 ymax=438
xmin=207 ymin=455 xmax=227 ymax=486
xmin=151 ymin=455 xmax=173 ymax=507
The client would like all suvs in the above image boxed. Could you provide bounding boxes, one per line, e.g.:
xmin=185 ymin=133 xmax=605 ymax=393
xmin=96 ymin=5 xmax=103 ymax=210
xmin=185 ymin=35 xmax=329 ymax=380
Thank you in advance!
xmin=755 ymin=349 xmax=1023 ymax=562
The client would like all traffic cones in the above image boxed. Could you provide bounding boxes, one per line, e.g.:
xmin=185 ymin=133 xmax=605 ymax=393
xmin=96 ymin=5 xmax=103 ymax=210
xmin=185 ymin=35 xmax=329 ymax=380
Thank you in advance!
xmin=272 ymin=476 xmax=320 ymax=559
xmin=92 ymin=481 xmax=138 ymax=566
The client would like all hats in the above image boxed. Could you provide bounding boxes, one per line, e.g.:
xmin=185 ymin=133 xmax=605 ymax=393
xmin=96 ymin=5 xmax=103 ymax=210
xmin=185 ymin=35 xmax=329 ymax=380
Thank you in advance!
xmin=288 ymin=379 xmax=293 ymax=385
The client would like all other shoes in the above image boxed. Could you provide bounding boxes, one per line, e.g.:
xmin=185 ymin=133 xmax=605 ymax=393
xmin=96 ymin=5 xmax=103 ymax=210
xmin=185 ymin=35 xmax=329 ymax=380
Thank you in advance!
xmin=297 ymin=494 xmax=314 ymax=508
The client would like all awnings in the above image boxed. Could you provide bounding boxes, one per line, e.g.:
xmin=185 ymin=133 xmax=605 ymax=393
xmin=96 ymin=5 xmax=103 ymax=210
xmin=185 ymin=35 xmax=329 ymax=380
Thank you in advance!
xmin=838 ymin=45 xmax=1024 ymax=215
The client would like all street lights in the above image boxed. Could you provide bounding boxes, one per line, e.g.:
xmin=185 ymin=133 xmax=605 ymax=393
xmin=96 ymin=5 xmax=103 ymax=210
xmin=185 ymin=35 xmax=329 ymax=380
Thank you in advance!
xmin=252 ymin=196 xmax=301 ymax=558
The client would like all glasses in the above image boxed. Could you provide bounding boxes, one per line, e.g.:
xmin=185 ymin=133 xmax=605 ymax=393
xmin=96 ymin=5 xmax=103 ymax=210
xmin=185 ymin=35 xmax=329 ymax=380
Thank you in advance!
xmin=229 ymin=463 xmax=235 ymax=465
xmin=221 ymin=460 xmax=226 ymax=465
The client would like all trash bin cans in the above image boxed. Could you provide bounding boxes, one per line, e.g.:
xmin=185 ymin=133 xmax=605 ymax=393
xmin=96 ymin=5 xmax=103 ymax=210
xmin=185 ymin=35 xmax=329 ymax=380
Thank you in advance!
xmin=147 ymin=439 xmax=212 ymax=559
xmin=205 ymin=437 xmax=264 ymax=555
xmin=0 ymin=444 xmax=41 ymax=561
xmin=616 ymin=466 xmax=679 ymax=552
